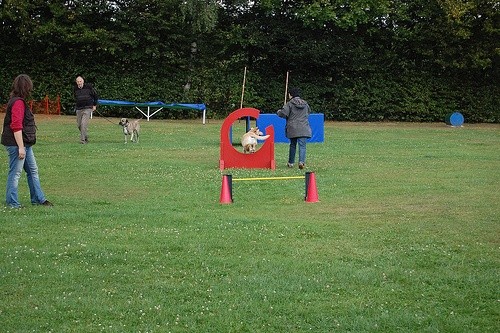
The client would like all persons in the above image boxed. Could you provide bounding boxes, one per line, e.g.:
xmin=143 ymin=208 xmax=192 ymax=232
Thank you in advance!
xmin=74 ymin=76 xmax=98 ymax=143
xmin=1 ymin=74 xmax=54 ymax=207
xmin=277 ymin=88 xmax=311 ymax=169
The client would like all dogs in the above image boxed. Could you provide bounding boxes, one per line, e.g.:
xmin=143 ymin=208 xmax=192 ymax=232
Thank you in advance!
xmin=241 ymin=127 xmax=270 ymax=153
xmin=119 ymin=118 xmax=142 ymax=145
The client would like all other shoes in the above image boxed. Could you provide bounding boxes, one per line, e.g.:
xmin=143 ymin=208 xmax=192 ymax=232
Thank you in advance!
xmin=287 ymin=162 xmax=294 ymax=168
xmin=298 ymin=162 xmax=304 ymax=168
xmin=85 ymin=139 xmax=89 ymax=144
xmin=80 ymin=140 xmax=86 ymax=144
xmin=43 ymin=201 xmax=53 ymax=206
